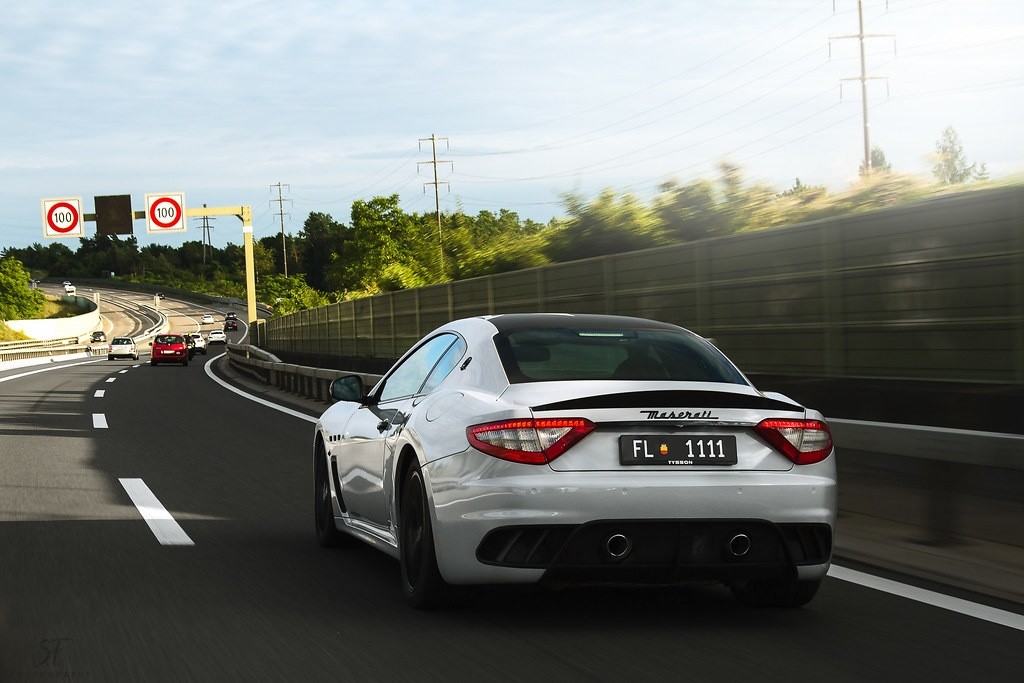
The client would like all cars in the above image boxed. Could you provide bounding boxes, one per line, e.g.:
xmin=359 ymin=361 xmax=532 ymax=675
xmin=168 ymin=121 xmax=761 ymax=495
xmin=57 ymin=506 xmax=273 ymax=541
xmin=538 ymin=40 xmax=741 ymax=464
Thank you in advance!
xmin=63 ymin=281 xmax=71 ymax=287
xmin=36 ymin=280 xmax=41 ymax=284
xmin=201 ymin=315 xmax=214 ymax=325
xmin=90 ymin=332 xmax=107 ymax=343
xmin=208 ymin=331 xmax=227 ymax=345
xmin=108 ymin=336 xmax=140 ymax=360
xmin=156 ymin=293 xmax=165 ymax=299
xmin=182 ymin=335 xmax=195 ymax=360
xmin=225 ymin=311 xmax=237 ymax=321
xmin=189 ymin=333 xmax=207 ymax=354
xmin=224 ymin=320 xmax=238 ymax=331
xmin=149 ymin=333 xmax=189 ymax=366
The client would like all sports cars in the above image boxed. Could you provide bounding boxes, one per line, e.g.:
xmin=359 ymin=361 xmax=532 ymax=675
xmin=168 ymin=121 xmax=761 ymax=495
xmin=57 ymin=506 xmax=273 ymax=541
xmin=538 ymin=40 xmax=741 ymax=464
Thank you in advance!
xmin=312 ymin=312 xmax=839 ymax=613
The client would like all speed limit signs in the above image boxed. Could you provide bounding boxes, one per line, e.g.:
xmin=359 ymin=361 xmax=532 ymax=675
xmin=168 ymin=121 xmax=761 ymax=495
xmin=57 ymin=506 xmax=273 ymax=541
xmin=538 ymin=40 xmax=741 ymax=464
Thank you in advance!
xmin=41 ymin=197 xmax=85 ymax=238
xmin=145 ymin=193 xmax=188 ymax=233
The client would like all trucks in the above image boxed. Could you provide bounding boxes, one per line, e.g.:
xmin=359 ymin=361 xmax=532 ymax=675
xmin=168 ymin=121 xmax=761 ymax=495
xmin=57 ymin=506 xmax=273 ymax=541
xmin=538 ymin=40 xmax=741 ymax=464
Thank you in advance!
xmin=65 ymin=286 xmax=76 ymax=296
